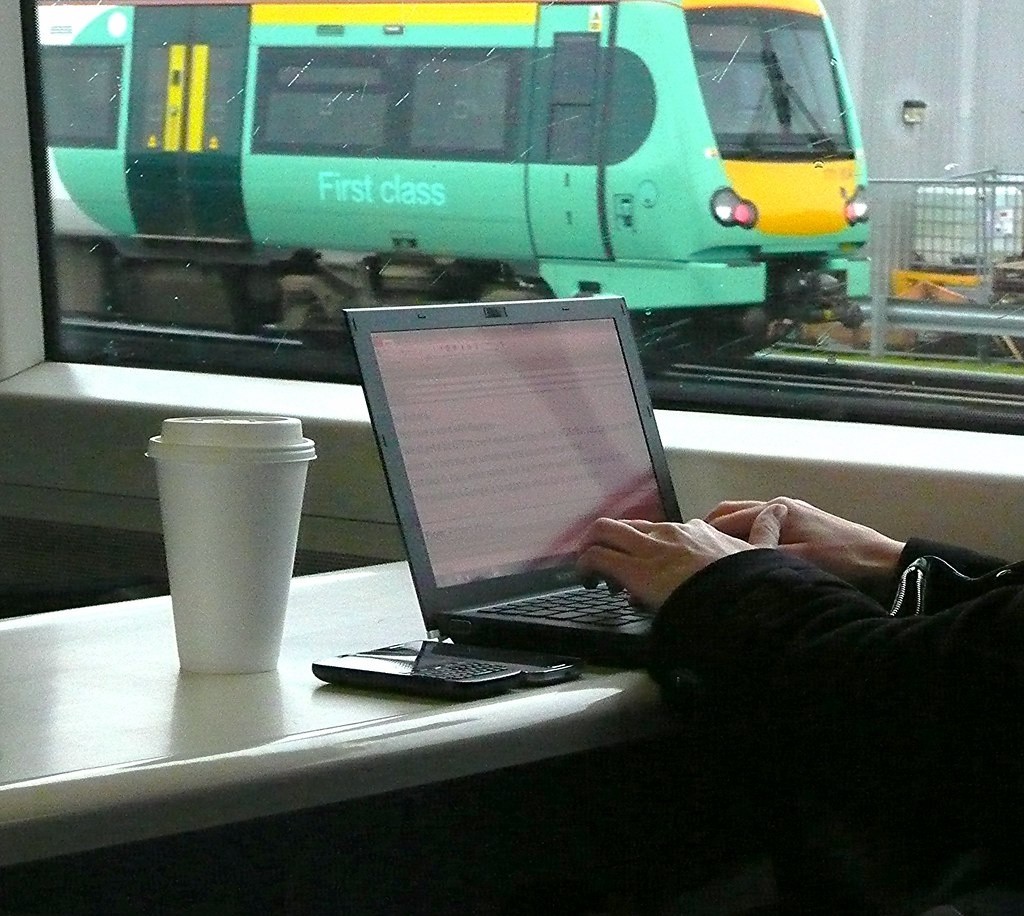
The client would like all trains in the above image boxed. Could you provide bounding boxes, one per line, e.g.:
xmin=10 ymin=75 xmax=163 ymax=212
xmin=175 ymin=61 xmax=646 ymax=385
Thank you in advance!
xmin=32 ymin=2 xmax=876 ymax=341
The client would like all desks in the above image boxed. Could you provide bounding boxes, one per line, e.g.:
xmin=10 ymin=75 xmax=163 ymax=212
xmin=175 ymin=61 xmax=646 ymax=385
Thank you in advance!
xmin=0 ymin=560 xmax=666 ymax=874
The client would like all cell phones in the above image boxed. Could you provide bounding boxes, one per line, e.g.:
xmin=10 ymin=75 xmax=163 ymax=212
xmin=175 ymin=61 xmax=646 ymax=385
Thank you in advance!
xmin=311 ymin=640 xmax=587 ymax=698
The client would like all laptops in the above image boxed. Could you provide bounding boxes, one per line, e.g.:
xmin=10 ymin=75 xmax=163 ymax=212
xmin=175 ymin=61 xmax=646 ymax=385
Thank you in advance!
xmin=340 ymin=296 xmax=686 ymax=666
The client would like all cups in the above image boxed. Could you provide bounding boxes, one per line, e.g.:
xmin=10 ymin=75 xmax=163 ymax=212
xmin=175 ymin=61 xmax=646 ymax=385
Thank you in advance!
xmin=145 ymin=416 xmax=317 ymax=676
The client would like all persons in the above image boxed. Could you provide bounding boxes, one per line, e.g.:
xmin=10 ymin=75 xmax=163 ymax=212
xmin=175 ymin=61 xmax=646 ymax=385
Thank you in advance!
xmin=577 ymin=493 xmax=1024 ymax=916
